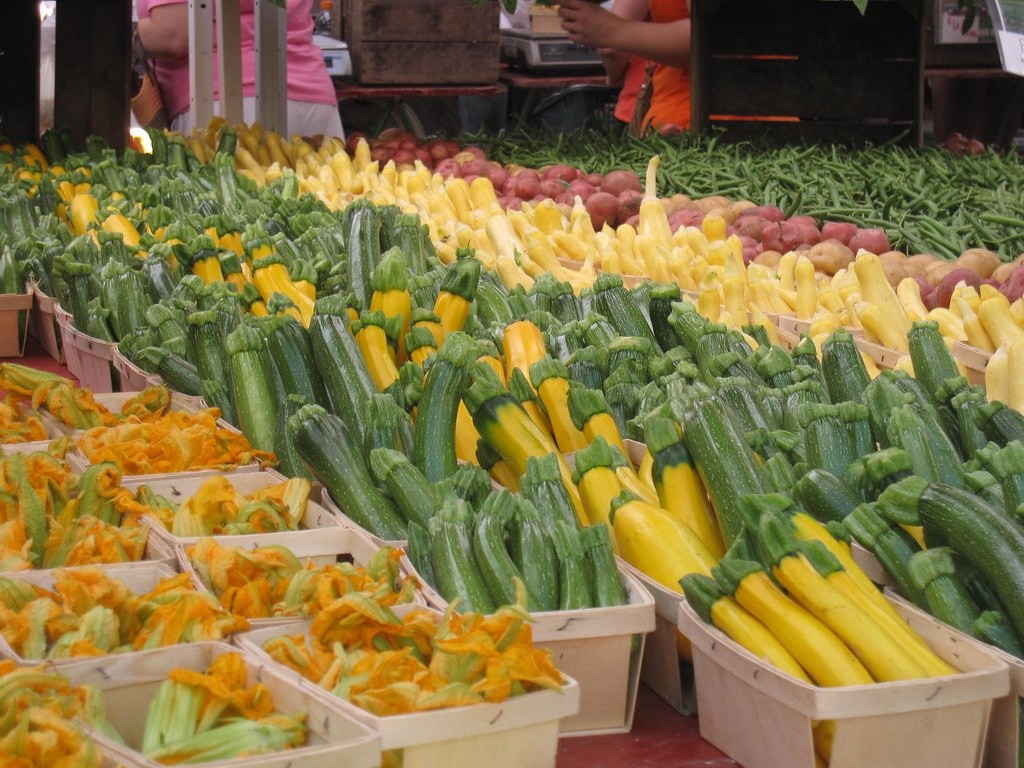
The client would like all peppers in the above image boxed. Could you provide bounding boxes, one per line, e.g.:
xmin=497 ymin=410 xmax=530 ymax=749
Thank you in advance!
xmin=427 ymin=115 xmax=1024 ymax=265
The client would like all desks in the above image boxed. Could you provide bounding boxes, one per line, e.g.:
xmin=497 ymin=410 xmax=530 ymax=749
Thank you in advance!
xmin=333 ymin=64 xmax=608 ymax=139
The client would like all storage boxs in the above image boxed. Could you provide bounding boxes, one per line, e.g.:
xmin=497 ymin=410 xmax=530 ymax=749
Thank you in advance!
xmin=0 ymin=261 xmax=1024 ymax=768
xmin=329 ymin=0 xmax=504 ymax=87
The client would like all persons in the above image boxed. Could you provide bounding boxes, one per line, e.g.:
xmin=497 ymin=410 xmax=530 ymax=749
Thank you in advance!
xmin=135 ymin=0 xmax=347 ymax=152
xmin=557 ymin=0 xmax=692 ymax=139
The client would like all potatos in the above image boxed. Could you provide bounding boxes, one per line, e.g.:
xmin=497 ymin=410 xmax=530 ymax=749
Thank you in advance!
xmin=308 ymin=133 xmax=1024 ymax=392
